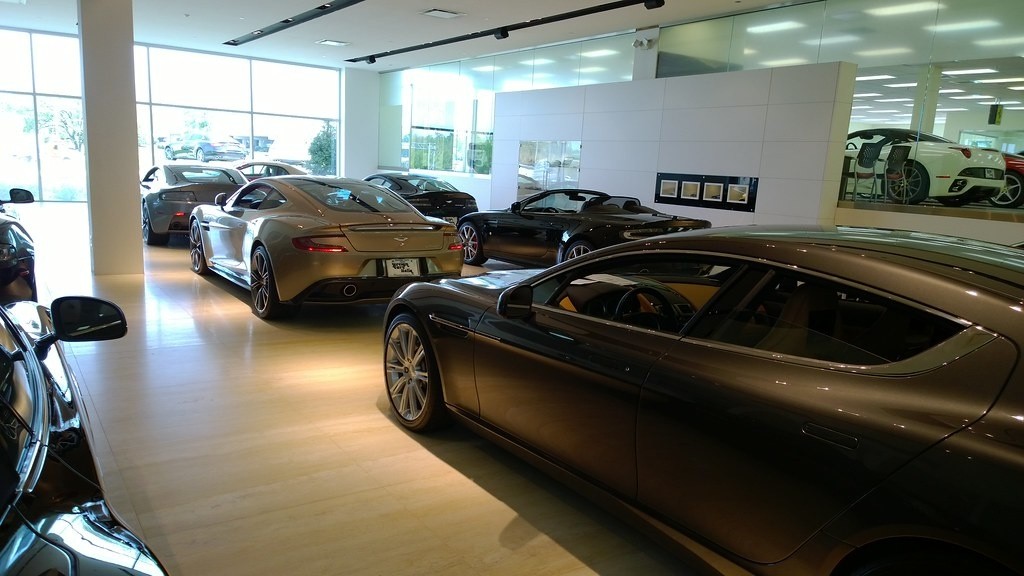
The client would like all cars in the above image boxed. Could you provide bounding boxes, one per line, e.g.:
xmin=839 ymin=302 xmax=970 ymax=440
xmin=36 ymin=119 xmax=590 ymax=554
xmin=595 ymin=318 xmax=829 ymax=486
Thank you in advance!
xmin=975 ymin=148 xmax=1024 ymax=209
xmin=360 ymin=172 xmax=478 ymax=222
xmin=380 ymin=221 xmax=1023 ymax=576
xmin=235 ymin=161 xmax=307 ymax=188
xmin=0 ymin=187 xmax=38 ymax=307
xmin=0 ymin=296 xmax=171 ymax=576
xmin=164 ymin=130 xmax=248 ymax=162
xmin=843 ymin=127 xmax=1007 ymax=207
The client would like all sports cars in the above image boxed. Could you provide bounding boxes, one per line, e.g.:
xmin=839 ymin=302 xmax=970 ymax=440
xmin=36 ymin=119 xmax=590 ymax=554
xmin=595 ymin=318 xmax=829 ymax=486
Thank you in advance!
xmin=139 ymin=163 xmax=253 ymax=247
xmin=188 ymin=174 xmax=467 ymax=321
xmin=455 ymin=187 xmax=714 ymax=271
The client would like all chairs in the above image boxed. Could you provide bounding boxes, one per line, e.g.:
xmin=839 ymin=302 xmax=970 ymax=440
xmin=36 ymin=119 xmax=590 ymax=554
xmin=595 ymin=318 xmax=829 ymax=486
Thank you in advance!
xmin=757 ymin=284 xmax=844 ymax=355
xmin=577 ymin=201 xmax=599 ymax=213
xmin=840 ymin=143 xmax=886 ymax=202
xmin=623 ymin=201 xmax=637 ymax=208
xmin=869 ymin=144 xmax=912 ymax=202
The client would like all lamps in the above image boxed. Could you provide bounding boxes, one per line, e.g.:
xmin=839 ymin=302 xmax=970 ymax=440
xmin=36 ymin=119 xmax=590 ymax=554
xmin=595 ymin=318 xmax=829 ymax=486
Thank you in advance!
xmin=644 ymin=0 xmax=665 ymax=9
xmin=367 ymin=56 xmax=376 ymax=64
xmin=495 ymin=27 xmax=509 ymax=39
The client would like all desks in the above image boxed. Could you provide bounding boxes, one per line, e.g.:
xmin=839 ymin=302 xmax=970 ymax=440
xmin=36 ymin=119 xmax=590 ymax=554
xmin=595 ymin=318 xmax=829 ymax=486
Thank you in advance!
xmin=838 ymin=150 xmax=860 ymax=200
